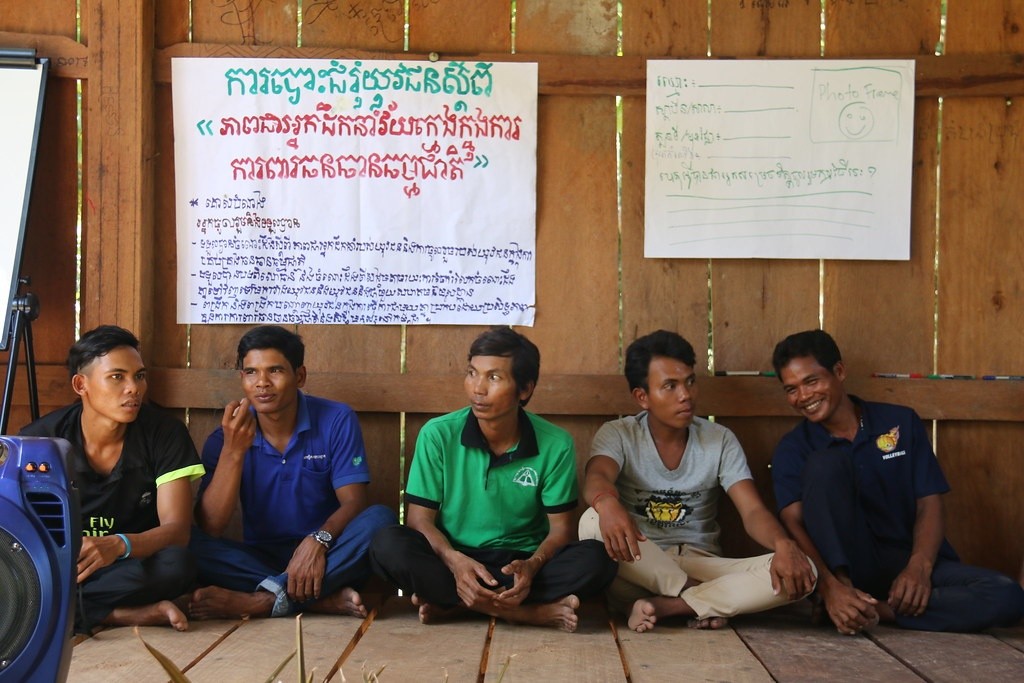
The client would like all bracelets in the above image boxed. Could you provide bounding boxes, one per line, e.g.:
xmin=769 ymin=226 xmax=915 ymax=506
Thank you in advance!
xmin=591 ymin=492 xmax=617 ymax=513
xmin=114 ymin=533 xmax=130 ymax=560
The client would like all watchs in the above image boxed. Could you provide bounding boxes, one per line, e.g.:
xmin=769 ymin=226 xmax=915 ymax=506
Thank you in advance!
xmin=309 ymin=531 xmax=333 ymax=548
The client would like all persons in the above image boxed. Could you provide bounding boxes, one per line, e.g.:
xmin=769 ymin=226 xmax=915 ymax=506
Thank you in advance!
xmin=770 ymin=331 xmax=1024 ymax=635
xmin=188 ymin=325 xmax=400 ymax=623
xmin=14 ymin=325 xmax=206 ymax=631
xmin=577 ymin=330 xmax=818 ymax=632
xmin=370 ymin=328 xmax=619 ymax=633
